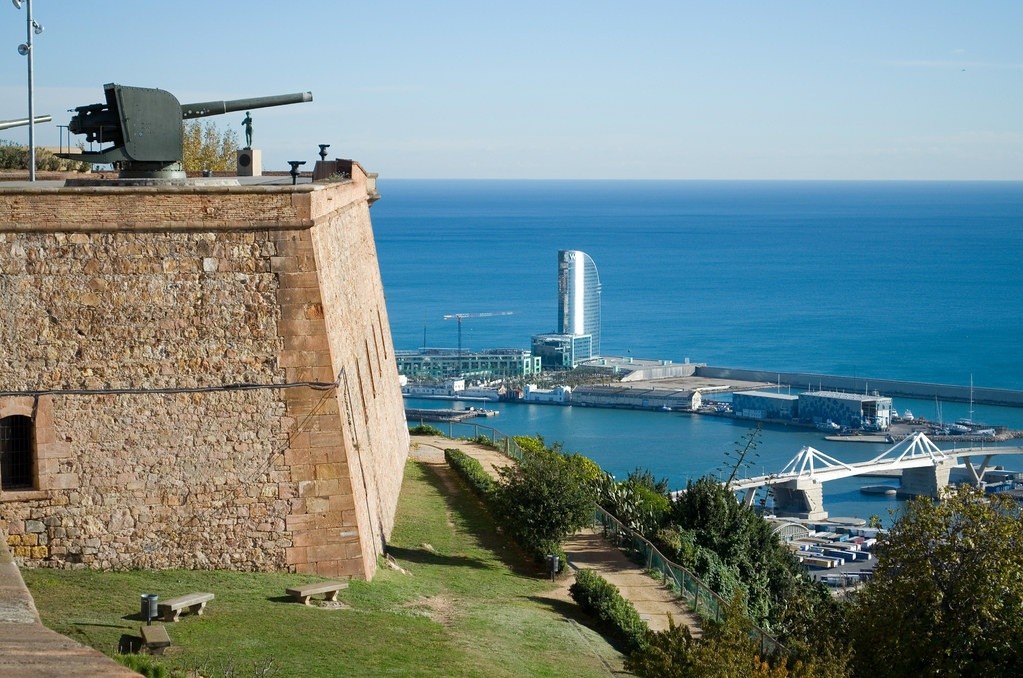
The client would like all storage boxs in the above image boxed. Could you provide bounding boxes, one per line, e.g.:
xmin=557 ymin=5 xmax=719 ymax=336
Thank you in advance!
xmin=787 ymin=519 xmax=889 ymax=588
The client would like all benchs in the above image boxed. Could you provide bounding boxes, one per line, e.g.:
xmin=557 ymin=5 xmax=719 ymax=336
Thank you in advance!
xmin=158 ymin=591 xmax=214 ymax=622
xmin=286 ymin=579 xmax=348 ymax=605
xmin=139 ymin=623 xmax=172 ymax=656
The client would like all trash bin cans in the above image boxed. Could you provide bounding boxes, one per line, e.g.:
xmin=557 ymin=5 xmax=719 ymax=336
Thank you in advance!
xmin=141 ymin=593 xmax=158 ymax=617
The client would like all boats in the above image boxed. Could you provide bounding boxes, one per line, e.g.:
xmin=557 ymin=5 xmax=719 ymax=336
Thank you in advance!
xmin=951 ymin=425 xmax=972 ymax=434
xmin=903 ymin=409 xmax=915 ymax=421
xmin=892 ymin=408 xmax=898 ymax=418
xmin=816 ymin=420 xmax=842 ymax=432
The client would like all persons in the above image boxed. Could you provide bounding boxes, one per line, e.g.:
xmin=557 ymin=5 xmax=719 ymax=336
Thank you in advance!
xmin=241 ymin=111 xmax=253 ymax=148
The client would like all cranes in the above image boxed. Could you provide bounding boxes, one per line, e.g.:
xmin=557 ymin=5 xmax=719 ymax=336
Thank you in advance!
xmin=443 ymin=310 xmax=522 ymax=358
